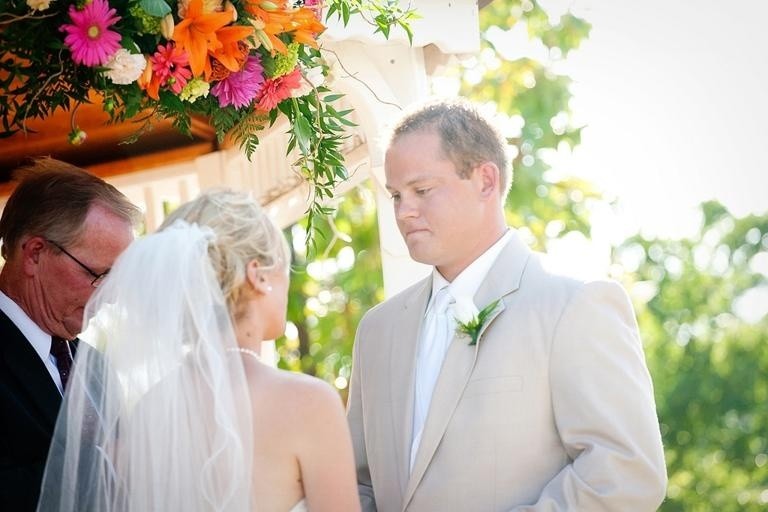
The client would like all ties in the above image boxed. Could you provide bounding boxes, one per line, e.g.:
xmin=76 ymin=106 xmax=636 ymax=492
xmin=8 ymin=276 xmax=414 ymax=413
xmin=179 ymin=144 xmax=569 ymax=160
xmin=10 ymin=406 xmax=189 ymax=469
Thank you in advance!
xmin=409 ymin=288 xmax=454 ymax=476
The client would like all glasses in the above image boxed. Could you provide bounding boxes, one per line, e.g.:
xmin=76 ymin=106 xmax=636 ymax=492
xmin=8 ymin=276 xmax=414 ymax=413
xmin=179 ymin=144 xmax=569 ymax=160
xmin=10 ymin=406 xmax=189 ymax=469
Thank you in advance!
xmin=22 ymin=236 xmax=109 ymax=289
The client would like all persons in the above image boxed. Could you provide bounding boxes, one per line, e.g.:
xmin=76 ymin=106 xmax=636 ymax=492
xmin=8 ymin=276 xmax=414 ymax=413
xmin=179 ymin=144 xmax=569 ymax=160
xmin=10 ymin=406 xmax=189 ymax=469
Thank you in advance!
xmin=342 ymin=102 xmax=670 ymax=512
xmin=36 ymin=186 xmax=363 ymax=511
xmin=0 ymin=153 xmax=146 ymax=509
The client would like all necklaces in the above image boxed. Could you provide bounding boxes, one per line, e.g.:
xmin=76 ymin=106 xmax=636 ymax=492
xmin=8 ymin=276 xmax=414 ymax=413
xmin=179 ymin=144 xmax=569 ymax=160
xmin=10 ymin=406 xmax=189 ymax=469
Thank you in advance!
xmin=226 ymin=347 xmax=264 ymax=363
